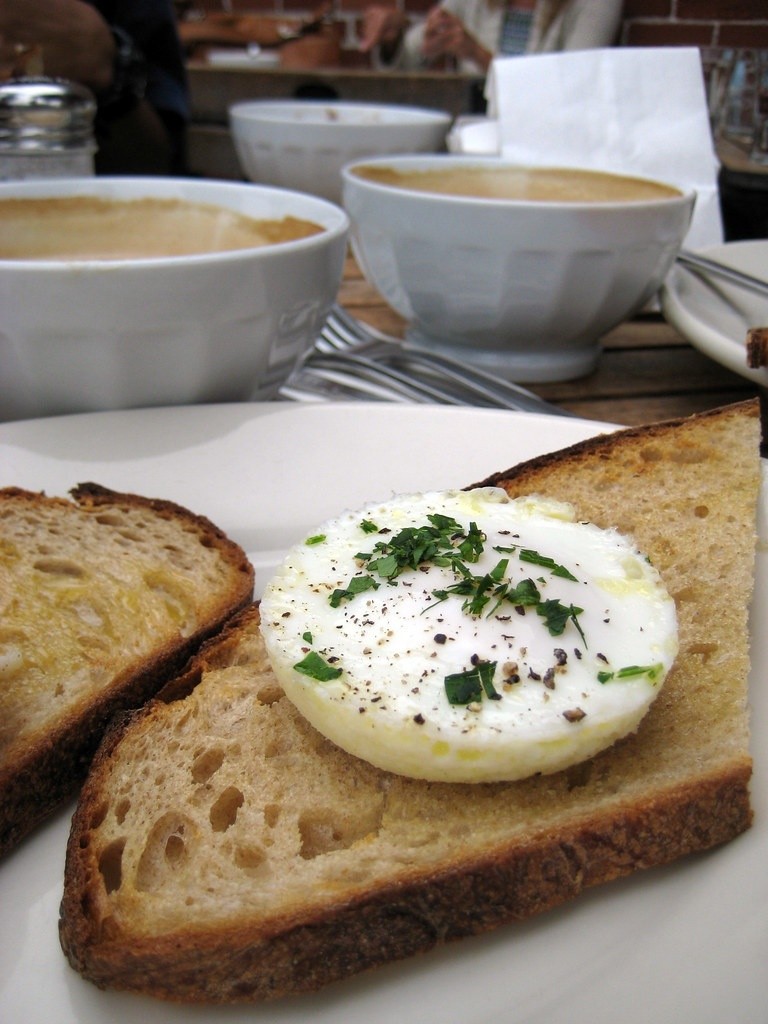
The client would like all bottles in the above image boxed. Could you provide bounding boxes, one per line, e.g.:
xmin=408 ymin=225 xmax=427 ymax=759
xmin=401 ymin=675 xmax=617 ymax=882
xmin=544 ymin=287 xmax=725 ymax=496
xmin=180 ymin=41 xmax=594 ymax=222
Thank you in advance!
xmin=0 ymin=82 xmax=98 ymax=179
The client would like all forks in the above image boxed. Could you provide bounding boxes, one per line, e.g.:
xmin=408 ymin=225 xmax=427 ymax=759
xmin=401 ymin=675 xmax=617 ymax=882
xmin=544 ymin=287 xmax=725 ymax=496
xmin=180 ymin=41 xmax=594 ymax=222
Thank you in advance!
xmin=316 ymin=304 xmax=575 ymax=418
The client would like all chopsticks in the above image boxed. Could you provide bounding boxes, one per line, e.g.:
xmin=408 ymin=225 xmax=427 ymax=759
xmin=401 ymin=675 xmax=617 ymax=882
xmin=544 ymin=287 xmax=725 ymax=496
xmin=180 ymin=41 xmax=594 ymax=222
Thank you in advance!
xmin=677 ymin=250 xmax=768 ymax=300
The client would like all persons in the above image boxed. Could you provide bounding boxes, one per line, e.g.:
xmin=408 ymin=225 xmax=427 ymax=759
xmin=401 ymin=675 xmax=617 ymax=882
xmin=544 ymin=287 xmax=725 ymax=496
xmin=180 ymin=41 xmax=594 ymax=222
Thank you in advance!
xmin=2 ymin=0 xmax=203 ymax=176
xmin=358 ymin=0 xmax=625 ymax=115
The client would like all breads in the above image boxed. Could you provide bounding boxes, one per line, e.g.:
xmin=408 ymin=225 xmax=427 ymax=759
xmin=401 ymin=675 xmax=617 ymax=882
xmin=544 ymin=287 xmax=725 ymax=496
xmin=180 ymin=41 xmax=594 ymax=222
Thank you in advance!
xmin=0 ymin=481 xmax=256 ymax=861
xmin=58 ymin=395 xmax=760 ymax=995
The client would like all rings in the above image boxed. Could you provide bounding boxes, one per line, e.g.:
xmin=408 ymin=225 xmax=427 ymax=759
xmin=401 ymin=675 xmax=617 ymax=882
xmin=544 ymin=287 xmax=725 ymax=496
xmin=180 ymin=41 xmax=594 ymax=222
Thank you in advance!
xmin=14 ymin=41 xmax=26 ymax=56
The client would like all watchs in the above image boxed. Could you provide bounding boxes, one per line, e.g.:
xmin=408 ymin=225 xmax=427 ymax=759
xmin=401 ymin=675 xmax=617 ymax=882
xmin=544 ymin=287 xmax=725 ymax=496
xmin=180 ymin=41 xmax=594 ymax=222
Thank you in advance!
xmin=76 ymin=27 xmax=149 ymax=123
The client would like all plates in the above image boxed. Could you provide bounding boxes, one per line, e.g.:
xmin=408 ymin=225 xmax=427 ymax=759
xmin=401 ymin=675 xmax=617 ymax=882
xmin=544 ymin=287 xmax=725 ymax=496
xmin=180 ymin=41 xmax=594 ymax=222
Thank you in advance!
xmin=1 ymin=404 xmax=768 ymax=1022
xmin=661 ymin=238 xmax=768 ymax=386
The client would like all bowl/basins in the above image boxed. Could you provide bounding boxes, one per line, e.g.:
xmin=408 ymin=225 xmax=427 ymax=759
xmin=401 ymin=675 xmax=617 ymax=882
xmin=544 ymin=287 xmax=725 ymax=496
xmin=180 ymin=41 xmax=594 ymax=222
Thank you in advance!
xmin=340 ymin=157 xmax=698 ymax=382
xmin=229 ymin=101 xmax=453 ymax=208
xmin=0 ymin=179 xmax=350 ymax=420
